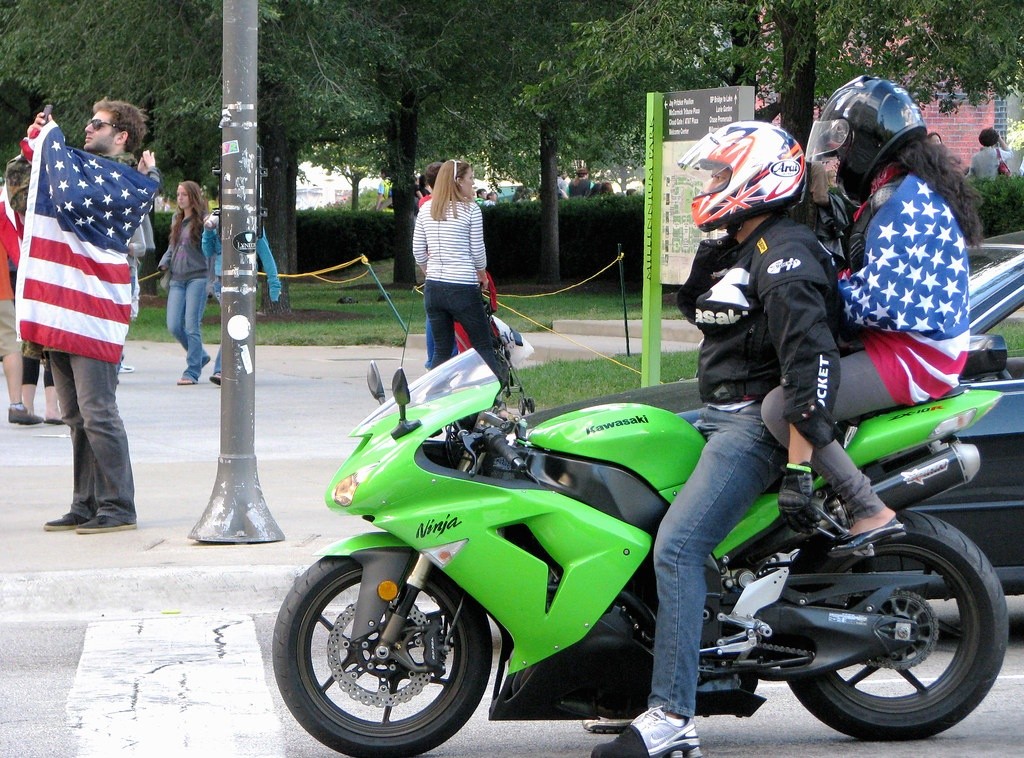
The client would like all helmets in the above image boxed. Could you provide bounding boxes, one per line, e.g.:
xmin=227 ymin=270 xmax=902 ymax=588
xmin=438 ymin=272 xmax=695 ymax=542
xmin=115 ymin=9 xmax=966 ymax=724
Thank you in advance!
xmin=805 ymin=75 xmax=927 ymax=205
xmin=678 ymin=120 xmax=807 ymax=234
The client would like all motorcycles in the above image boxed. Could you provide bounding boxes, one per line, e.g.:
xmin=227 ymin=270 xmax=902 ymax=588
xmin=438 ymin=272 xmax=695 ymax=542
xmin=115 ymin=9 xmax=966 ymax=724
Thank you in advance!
xmin=268 ymin=347 xmax=1010 ymax=758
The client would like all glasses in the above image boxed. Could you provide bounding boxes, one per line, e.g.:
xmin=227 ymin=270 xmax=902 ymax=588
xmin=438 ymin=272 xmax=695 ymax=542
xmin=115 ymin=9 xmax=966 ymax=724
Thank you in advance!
xmin=86 ymin=118 xmax=124 ymax=131
xmin=449 ymin=159 xmax=461 ymax=182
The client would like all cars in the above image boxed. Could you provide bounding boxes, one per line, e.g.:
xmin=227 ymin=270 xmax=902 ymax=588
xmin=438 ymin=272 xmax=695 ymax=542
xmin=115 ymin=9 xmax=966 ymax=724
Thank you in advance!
xmin=524 ymin=231 xmax=1024 ymax=598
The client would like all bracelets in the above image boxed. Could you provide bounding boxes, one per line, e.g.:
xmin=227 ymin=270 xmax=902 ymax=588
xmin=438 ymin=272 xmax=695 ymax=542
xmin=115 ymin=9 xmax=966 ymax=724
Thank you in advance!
xmin=785 ymin=463 xmax=811 ymax=472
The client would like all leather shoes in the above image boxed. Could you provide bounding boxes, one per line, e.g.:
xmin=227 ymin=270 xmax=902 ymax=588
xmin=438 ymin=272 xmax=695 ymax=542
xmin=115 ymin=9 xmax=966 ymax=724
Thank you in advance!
xmin=828 ymin=516 xmax=905 ymax=556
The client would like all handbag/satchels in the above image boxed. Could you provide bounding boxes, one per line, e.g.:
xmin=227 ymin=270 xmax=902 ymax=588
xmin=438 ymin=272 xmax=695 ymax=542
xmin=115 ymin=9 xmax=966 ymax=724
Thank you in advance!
xmin=996 ymin=148 xmax=1011 ymax=177
xmin=159 ymin=269 xmax=172 ymax=293
xmin=490 ymin=315 xmax=534 ymax=369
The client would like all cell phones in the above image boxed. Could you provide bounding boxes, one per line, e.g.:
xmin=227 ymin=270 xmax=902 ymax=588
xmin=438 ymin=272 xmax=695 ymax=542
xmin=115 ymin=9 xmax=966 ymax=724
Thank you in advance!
xmin=42 ymin=105 xmax=53 ymax=126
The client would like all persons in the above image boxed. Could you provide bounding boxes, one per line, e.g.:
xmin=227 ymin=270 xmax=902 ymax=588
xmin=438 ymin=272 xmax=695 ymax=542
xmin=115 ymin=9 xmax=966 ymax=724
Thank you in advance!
xmin=375 ymin=167 xmax=498 ymax=366
xmin=158 ymin=181 xmax=211 ymax=386
xmin=591 ymin=119 xmax=840 ymax=758
xmin=927 ymin=133 xmax=941 ymax=145
xmin=0 ymin=98 xmax=160 ymax=534
xmin=200 ymin=206 xmax=281 ymax=386
xmin=760 ymin=76 xmax=983 ymax=557
xmin=557 ymin=167 xmax=613 ymax=200
xmin=967 ymin=127 xmax=1013 ymax=179
xmin=411 ymin=159 xmax=499 ymax=376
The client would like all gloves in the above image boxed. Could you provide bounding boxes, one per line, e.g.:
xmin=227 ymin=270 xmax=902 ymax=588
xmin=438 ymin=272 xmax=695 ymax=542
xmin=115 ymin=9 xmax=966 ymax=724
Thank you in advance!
xmin=779 ymin=463 xmax=821 ymax=535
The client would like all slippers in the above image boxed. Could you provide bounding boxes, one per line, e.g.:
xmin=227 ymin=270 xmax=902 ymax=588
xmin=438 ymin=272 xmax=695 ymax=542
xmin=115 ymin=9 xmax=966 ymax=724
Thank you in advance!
xmin=42 ymin=417 xmax=64 ymax=424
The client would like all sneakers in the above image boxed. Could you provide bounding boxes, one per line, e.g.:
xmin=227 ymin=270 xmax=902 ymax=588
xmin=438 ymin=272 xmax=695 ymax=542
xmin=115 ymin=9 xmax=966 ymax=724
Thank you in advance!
xmin=583 ymin=717 xmax=637 ymax=735
xmin=8 ymin=404 xmax=44 ymax=424
xmin=591 ymin=704 xmax=701 ymax=758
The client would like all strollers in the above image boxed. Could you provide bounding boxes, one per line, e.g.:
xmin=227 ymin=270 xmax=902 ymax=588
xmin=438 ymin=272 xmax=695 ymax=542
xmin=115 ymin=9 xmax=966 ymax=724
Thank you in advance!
xmin=452 ymin=271 xmax=537 ymax=417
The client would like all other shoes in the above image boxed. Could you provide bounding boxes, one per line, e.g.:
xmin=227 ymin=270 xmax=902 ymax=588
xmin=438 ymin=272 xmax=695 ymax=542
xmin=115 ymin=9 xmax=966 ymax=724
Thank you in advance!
xmin=43 ymin=512 xmax=88 ymax=532
xmin=209 ymin=372 xmax=221 ymax=386
xmin=201 ymin=356 xmax=210 ymax=368
xmin=178 ymin=376 xmax=192 ymax=385
xmin=76 ymin=515 xmax=137 ymax=533
